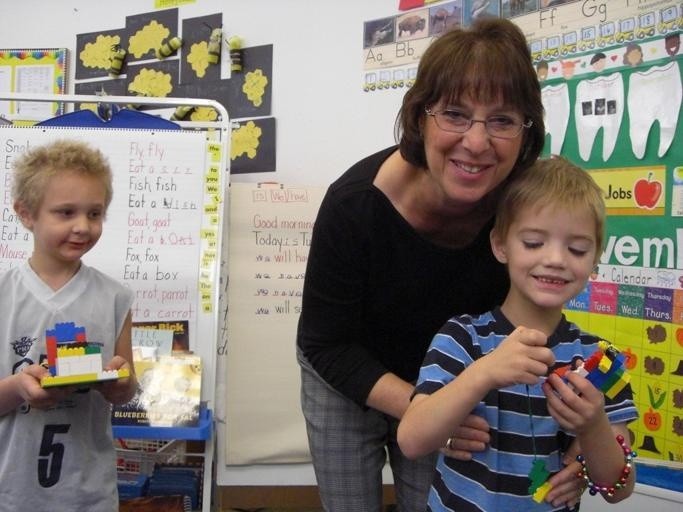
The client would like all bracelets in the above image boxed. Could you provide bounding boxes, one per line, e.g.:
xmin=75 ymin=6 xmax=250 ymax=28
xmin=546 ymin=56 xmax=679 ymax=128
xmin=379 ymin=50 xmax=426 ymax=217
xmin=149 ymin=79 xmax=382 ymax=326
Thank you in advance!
xmin=575 ymin=434 xmax=636 ymax=499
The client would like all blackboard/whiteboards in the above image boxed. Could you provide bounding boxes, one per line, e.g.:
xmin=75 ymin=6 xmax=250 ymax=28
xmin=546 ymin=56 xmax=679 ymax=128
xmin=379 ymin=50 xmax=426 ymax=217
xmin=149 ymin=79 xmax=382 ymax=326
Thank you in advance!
xmin=0 ymin=49 xmax=66 ymax=126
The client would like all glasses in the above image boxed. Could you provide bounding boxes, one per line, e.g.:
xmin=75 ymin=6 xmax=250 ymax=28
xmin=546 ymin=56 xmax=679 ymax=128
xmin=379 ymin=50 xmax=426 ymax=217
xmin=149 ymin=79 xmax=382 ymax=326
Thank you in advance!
xmin=423 ymin=107 xmax=534 ymax=140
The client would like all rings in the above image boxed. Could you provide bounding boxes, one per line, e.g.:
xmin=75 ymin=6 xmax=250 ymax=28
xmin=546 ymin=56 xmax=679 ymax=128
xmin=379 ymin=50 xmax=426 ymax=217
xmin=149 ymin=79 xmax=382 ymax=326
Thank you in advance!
xmin=446 ymin=438 xmax=453 ymax=449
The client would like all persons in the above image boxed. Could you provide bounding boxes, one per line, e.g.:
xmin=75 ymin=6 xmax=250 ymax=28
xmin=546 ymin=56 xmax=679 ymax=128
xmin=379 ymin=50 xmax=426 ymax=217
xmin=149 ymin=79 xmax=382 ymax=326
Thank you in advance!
xmin=396 ymin=158 xmax=637 ymax=511
xmin=2 ymin=139 xmax=140 ymax=511
xmin=295 ymin=13 xmax=545 ymax=511
